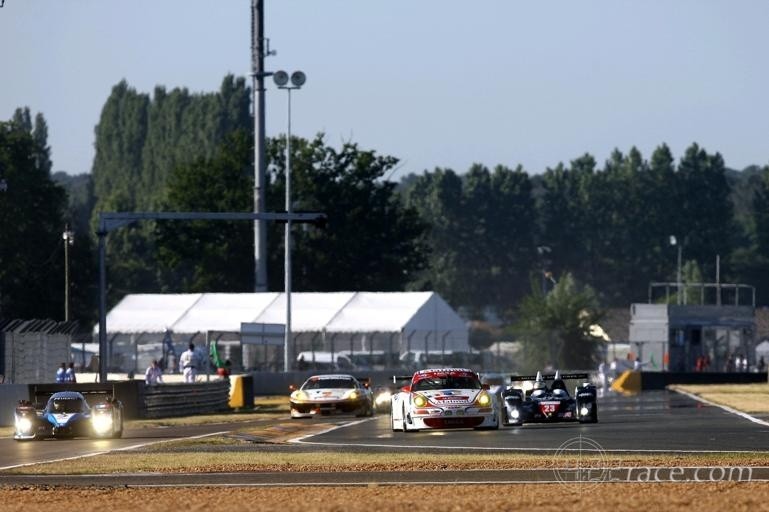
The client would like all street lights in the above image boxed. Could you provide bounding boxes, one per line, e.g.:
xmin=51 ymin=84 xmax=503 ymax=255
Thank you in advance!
xmin=60 ymin=225 xmax=75 ymax=324
xmin=273 ymin=70 xmax=304 ymax=374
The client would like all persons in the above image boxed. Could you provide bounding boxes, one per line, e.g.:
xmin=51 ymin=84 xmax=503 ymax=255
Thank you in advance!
xmin=65 ymin=362 xmax=76 ymax=384
xmin=599 ymin=357 xmax=647 ymax=392
xmin=166 ymin=350 xmax=176 ymax=374
xmin=145 ymin=359 xmax=162 ymax=385
xmin=56 ymin=362 xmax=66 ymax=383
xmin=53 ymin=400 xmax=64 ymax=409
xmin=695 ymin=353 xmax=765 ymax=373
xmin=179 ymin=343 xmax=201 ymax=383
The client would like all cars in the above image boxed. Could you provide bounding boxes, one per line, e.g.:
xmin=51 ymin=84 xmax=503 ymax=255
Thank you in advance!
xmin=500 ymin=372 xmax=597 ymax=428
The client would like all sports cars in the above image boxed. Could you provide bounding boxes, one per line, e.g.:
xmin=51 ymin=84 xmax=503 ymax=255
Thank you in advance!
xmin=288 ymin=373 xmax=377 ymax=420
xmin=389 ymin=368 xmax=501 ymax=433
xmin=12 ymin=391 xmax=124 ymax=443
